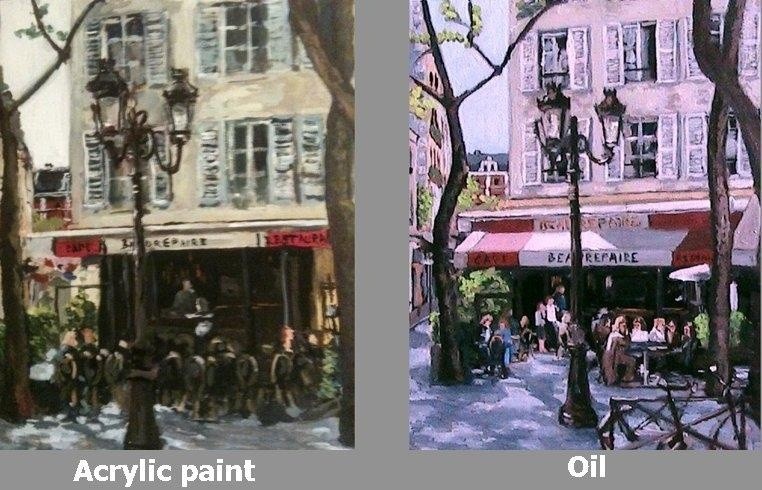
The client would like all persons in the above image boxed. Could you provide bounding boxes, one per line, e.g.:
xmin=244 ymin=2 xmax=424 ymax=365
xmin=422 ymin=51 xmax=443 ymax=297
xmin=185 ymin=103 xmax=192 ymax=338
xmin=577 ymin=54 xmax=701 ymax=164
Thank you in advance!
xmin=477 ymin=284 xmax=701 ymax=381
xmin=53 ymin=275 xmax=298 ymax=425
